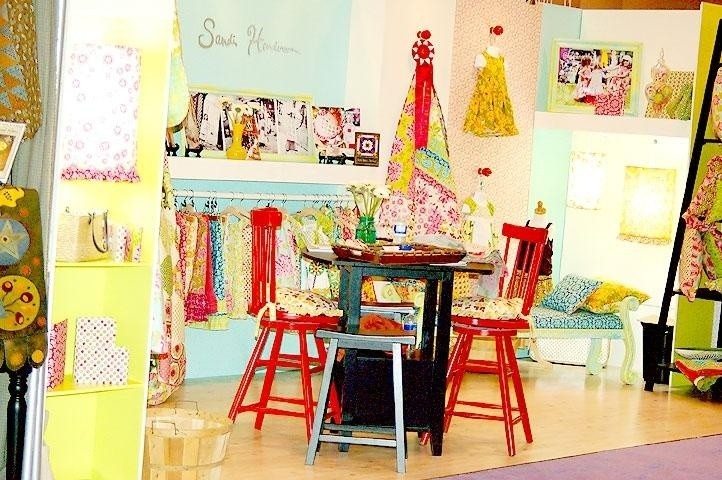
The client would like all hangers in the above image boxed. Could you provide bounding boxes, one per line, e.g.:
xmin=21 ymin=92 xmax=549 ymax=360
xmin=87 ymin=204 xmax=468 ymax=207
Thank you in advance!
xmin=479 ymin=171 xmax=485 ymax=196
xmin=170 ymin=188 xmax=353 ymax=215
xmin=491 ymin=27 xmax=498 ymax=49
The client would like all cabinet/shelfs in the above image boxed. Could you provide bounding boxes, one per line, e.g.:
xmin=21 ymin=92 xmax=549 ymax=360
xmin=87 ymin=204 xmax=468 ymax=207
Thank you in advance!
xmin=46 ymin=165 xmax=149 ymax=400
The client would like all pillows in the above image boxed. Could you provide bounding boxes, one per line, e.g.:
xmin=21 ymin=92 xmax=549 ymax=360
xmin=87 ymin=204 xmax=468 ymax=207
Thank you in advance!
xmin=538 ymin=272 xmax=604 ymax=316
xmin=578 ymin=274 xmax=651 ymax=316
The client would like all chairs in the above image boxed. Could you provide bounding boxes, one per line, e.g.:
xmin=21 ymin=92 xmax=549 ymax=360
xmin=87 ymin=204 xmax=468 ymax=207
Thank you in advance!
xmin=443 ymin=221 xmax=550 ymax=391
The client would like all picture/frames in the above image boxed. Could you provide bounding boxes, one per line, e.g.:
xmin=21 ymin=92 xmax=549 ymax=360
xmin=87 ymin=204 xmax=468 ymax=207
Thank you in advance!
xmin=0 ymin=121 xmax=27 ymax=187
xmin=546 ymin=36 xmax=644 ymax=118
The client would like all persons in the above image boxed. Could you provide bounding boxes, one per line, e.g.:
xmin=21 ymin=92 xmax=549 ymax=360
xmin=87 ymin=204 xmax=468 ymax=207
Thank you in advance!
xmin=463 ymin=46 xmax=520 ymax=138
xmin=572 ymin=53 xmax=633 ymax=114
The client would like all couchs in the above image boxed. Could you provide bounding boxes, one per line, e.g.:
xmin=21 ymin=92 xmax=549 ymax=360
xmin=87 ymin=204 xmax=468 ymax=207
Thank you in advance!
xmin=449 ymin=271 xmax=642 ymax=386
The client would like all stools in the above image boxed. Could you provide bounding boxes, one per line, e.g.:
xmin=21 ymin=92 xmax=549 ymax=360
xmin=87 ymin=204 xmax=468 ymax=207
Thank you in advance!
xmin=305 ymin=321 xmax=417 ymax=473
xmin=331 ymin=294 xmax=415 ymax=330
xmin=228 ymin=306 xmax=343 ymax=446
xmin=417 ymin=324 xmax=535 ymax=458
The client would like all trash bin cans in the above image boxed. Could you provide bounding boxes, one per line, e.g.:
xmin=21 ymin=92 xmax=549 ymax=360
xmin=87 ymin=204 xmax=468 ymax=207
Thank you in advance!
xmin=640 ymin=320 xmax=673 ymax=386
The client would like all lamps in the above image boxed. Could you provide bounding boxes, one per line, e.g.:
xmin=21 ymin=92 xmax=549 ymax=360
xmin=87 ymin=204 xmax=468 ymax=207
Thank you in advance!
xmin=620 ymin=166 xmax=678 ymax=247
xmin=565 ymin=150 xmax=607 ymax=210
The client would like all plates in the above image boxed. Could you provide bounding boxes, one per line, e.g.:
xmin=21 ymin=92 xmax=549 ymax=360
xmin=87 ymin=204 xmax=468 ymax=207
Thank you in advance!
xmin=673 ymin=347 xmax=722 ymax=361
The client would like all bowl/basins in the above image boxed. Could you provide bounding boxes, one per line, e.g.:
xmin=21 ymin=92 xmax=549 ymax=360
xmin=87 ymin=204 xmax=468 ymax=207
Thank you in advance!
xmin=142 ymin=399 xmax=235 ymax=467
xmin=142 ymin=463 xmax=222 ymax=479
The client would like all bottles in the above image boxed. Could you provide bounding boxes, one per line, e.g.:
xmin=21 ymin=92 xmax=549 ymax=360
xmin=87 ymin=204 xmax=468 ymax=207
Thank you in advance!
xmin=403 ymin=310 xmax=418 ymax=350
xmin=394 ymin=202 xmax=407 ymax=243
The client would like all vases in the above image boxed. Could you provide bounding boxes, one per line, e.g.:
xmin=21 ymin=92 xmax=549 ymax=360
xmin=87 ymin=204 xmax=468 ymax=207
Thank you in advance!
xmin=228 ymin=122 xmax=250 ymax=159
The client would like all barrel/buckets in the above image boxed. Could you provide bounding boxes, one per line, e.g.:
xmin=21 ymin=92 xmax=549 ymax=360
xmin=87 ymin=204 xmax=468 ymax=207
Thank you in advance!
xmin=142 ymin=399 xmax=233 ymax=480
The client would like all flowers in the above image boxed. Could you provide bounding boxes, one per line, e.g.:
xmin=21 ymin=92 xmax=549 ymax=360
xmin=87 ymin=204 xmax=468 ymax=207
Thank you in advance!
xmin=217 ymin=97 xmax=260 ymax=122
